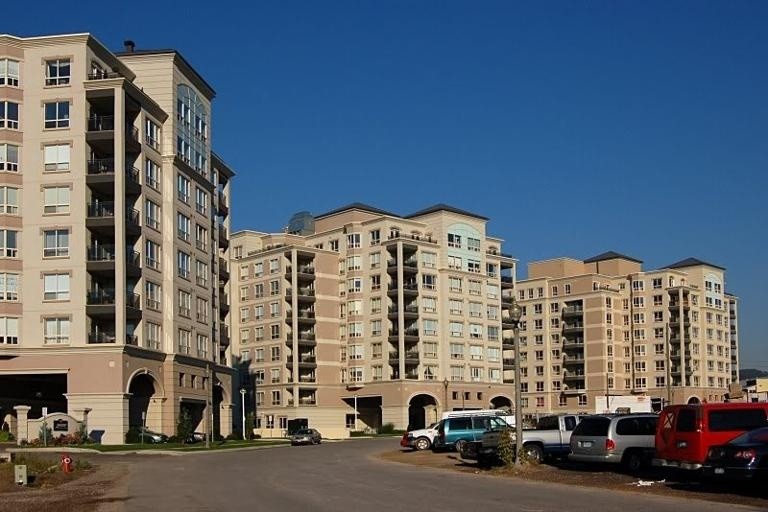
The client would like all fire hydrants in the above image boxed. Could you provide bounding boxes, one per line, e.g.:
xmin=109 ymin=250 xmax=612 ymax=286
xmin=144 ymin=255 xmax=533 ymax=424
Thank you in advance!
xmin=62 ymin=452 xmax=74 ymax=473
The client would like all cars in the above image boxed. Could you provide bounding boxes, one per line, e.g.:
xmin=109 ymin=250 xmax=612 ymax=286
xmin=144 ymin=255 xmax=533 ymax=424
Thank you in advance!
xmin=292 ymin=429 xmax=321 ymax=445
xmin=400 ymin=403 xmax=534 ymax=458
xmin=702 ymin=427 xmax=768 ymax=497
xmin=132 ymin=426 xmax=205 ymax=444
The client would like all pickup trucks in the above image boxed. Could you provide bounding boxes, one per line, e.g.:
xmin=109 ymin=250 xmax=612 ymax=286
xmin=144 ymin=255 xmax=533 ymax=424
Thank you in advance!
xmin=481 ymin=414 xmax=591 ymax=465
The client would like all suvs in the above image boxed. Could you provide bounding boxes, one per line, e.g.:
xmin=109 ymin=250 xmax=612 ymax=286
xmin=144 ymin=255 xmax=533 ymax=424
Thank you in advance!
xmin=567 ymin=412 xmax=661 ymax=475
xmin=654 ymin=400 xmax=768 ymax=482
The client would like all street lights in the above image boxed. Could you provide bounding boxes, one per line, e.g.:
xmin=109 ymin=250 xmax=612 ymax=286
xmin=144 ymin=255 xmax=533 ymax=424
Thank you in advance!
xmin=353 ymin=393 xmax=360 ymax=430
xmin=444 ymin=377 xmax=448 ymax=411
xmin=239 ymin=388 xmax=247 ymax=440
xmin=509 ymin=295 xmax=523 ymax=465
xmin=742 ymin=388 xmax=749 ymax=403
